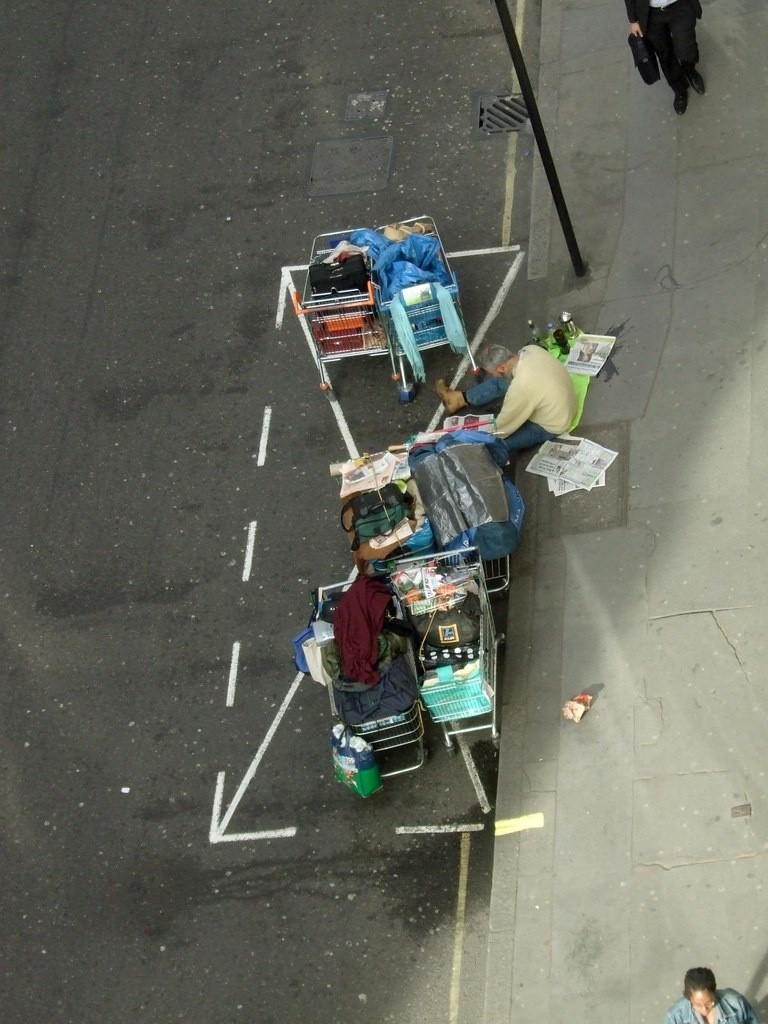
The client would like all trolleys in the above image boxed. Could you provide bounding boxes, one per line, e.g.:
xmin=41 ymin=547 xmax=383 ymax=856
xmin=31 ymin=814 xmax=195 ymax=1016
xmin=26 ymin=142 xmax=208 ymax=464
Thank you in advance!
xmin=373 ymin=213 xmax=486 ymax=403
xmin=328 ymin=419 xmax=501 ymax=778
xmin=293 ymin=227 xmax=403 ymax=402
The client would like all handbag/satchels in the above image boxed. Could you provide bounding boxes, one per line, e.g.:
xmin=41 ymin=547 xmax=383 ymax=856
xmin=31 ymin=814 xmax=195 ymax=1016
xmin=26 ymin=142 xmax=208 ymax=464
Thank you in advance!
xmin=627 ymin=33 xmax=661 ymax=84
xmin=341 ymin=483 xmax=434 ymax=574
xmin=292 ymin=575 xmax=483 ymax=799
xmin=309 ymin=240 xmax=378 ymax=323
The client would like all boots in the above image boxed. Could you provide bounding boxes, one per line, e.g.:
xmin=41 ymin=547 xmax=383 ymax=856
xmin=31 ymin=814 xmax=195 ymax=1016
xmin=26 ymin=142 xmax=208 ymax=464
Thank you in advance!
xmin=435 ymin=378 xmax=468 ymax=416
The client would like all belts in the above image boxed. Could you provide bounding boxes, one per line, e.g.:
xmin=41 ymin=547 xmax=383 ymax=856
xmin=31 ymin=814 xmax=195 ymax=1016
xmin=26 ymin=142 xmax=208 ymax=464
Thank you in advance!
xmin=650 ymin=0 xmax=680 ymax=13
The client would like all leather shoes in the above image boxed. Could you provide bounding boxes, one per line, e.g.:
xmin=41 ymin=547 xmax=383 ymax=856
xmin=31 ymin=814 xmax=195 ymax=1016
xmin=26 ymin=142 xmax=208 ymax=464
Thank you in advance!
xmin=681 ymin=64 xmax=705 ymax=95
xmin=673 ymin=77 xmax=690 ymax=114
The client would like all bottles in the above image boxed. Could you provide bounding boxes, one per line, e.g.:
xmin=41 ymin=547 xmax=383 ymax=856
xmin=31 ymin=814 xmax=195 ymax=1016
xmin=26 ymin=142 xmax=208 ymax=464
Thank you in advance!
xmin=547 ymin=324 xmax=556 ymax=345
xmin=528 ymin=320 xmax=550 ymax=354
xmin=560 ymin=312 xmax=577 ymax=339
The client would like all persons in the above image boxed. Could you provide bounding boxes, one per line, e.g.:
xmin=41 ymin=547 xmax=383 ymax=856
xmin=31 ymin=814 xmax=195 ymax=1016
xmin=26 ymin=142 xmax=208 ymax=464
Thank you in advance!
xmin=434 ymin=343 xmax=579 ymax=455
xmin=664 ymin=967 xmax=761 ymax=1024
xmin=624 ymin=0 xmax=707 ymax=112
